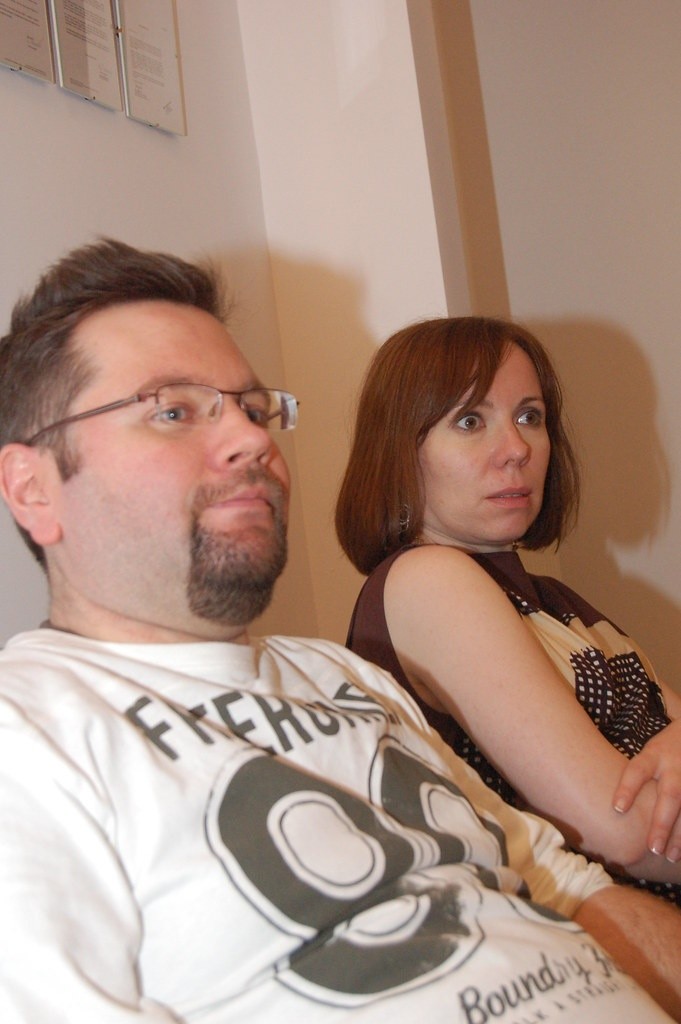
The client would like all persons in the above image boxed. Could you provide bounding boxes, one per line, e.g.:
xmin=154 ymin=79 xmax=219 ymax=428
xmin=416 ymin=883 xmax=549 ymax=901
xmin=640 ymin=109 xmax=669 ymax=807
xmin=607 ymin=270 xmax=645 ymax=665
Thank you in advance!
xmin=335 ymin=317 xmax=681 ymax=909
xmin=0 ymin=241 xmax=680 ymax=1024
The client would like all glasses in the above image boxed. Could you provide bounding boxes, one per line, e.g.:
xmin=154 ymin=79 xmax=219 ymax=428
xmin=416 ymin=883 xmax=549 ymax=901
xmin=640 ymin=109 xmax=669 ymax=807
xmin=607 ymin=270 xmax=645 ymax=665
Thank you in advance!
xmin=23 ymin=382 xmax=300 ymax=446
